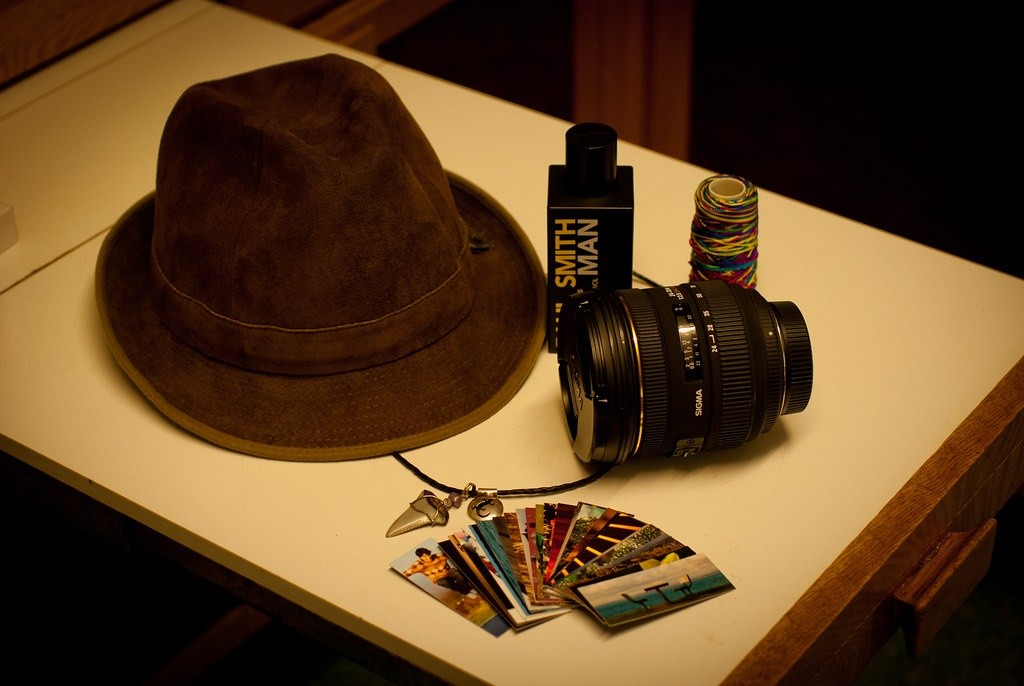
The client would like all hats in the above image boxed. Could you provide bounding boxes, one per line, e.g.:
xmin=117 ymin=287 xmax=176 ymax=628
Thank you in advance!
xmin=93 ymin=53 xmax=549 ymax=462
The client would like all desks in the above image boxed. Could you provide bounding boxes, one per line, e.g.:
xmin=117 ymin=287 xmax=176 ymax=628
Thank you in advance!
xmin=0 ymin=0 xmax=1024 ymax=686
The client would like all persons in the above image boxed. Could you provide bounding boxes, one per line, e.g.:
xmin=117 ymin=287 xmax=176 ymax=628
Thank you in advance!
xmin=403 ymin=548 xmax=477 ymax=600
